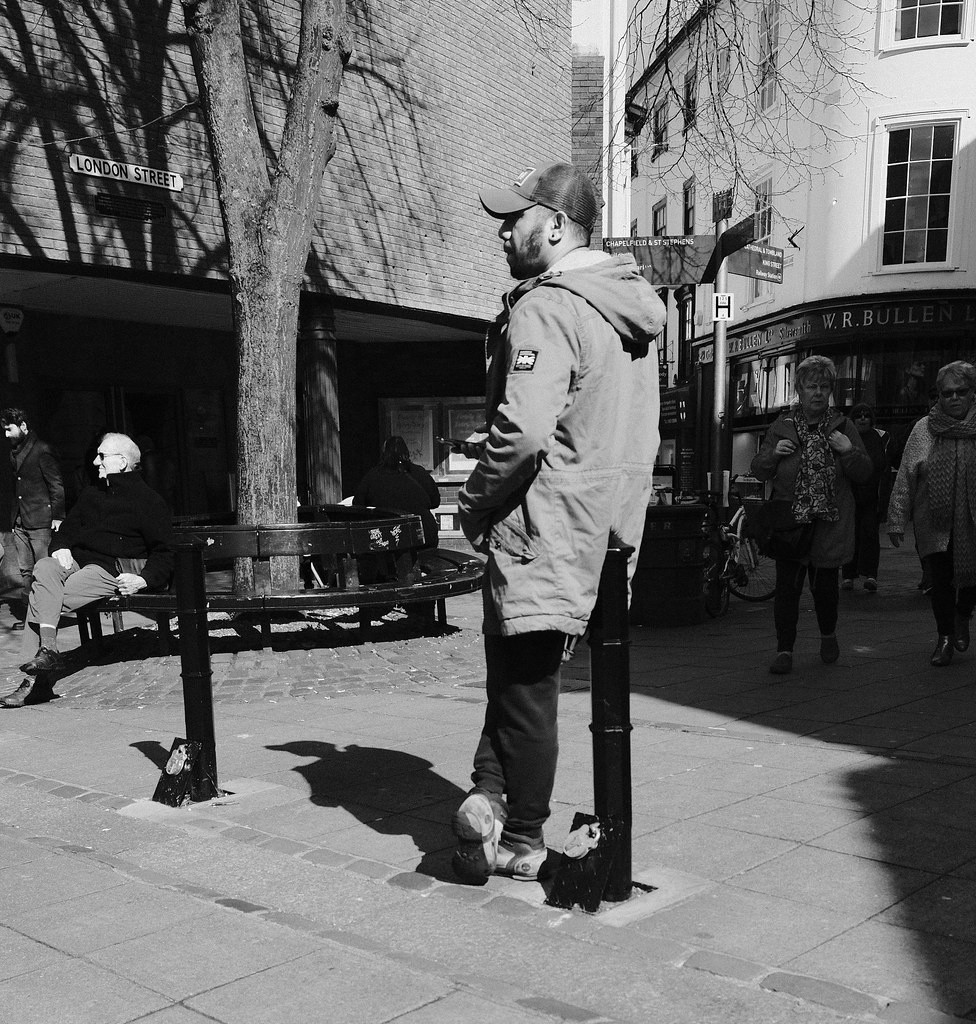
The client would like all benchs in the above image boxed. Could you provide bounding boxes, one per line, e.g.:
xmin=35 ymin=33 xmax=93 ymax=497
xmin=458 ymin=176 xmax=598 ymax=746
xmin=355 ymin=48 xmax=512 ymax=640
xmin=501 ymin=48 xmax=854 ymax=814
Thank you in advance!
xmin=72 ymin=543 xmax=488 ymax=652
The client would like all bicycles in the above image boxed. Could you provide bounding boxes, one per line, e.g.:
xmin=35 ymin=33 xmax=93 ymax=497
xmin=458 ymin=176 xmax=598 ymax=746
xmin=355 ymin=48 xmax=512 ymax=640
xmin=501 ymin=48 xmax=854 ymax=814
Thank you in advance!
xmin=654 ymin=486 xmax=721 ymax=506
xmin=700 ymin=474 xmax=777 ymax=619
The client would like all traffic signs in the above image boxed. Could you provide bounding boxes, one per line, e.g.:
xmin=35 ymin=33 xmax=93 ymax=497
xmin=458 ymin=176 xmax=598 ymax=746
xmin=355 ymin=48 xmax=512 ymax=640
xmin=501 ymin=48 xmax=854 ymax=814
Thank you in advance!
xmin=602 ymin=235 xmax=717 ymax=285
xmin=723 ymin=213 xmax=757 ymax=257
xmin=728 ymin=241 xmax=784 ymax=284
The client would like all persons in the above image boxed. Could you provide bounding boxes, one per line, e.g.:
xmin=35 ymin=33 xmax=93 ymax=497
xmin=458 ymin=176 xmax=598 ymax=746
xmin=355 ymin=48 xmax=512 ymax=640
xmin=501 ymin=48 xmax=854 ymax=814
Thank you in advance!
xmin=841 ymin=383 xmax=940 ymax=596
xmin=0 ymin=432 xmax=174 ymax=707
xmin=749 ymin=355 xmax=873 ymax=675
xmin=886 ymin=360 xmax=976 ymax=666
xmin=453 ymin=161 xmax=671 ymax=881
xmin=352 ymin=435 xmax=441 ymax=626
xmin=0 ymin=406 xmax=66 ymax=630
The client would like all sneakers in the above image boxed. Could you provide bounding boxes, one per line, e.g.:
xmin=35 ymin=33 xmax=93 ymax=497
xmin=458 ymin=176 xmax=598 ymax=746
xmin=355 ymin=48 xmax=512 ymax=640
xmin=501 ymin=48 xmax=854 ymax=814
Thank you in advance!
xmin=449 ymin=794 xmax=502 ymax=886
xmin=496 ymin=829 xmax=548 ymax=882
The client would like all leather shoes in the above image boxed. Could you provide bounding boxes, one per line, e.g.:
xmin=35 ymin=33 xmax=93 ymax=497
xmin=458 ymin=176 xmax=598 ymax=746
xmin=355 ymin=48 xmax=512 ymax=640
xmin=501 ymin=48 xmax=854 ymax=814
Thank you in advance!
xmin=0 ymin=678 xmax=35 ymax=707
xmin=25 ymin=646 xmax=66 ymax=675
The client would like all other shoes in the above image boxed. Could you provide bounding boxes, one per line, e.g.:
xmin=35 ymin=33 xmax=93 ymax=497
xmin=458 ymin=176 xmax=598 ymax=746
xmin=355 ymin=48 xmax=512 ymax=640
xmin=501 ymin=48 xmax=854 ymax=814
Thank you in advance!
xmin=769 ymin=649 xmax=793 ymax=673
xmin=955 ymin=608 xmax=970 ymax=652
xmin=819 ymin=633 xmax=839 ymax=663
xmin=13 ymin=622 xmax=24 ymax=629
xmin=841 ymin=578 xmax=853 ymax=589
xmin=931 ymin=633 xmax=954 ymax=665
xmin=863 ymin=574 xmax=877 ymax=593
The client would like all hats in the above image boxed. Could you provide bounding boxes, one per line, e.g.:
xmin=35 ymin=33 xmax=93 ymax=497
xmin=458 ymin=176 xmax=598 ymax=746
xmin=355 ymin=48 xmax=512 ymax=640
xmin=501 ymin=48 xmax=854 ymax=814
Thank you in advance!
xmin=478 ymin=163 xmax=597 ymax=233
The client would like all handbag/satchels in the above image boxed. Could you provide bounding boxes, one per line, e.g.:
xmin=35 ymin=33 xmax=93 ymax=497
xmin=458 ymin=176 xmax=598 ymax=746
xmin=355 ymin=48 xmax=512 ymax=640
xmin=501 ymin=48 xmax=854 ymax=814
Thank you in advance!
xmin=743 ymin=501 xmax=801 ymax=561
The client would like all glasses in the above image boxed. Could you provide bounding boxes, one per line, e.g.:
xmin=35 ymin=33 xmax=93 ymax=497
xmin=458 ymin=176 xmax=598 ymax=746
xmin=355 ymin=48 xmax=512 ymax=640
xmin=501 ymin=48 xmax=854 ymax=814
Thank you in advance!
xmin=98 ymin=452 xmax=127 ymax=461
xmin=940 ymin=387 xmax=970 ymax=398
